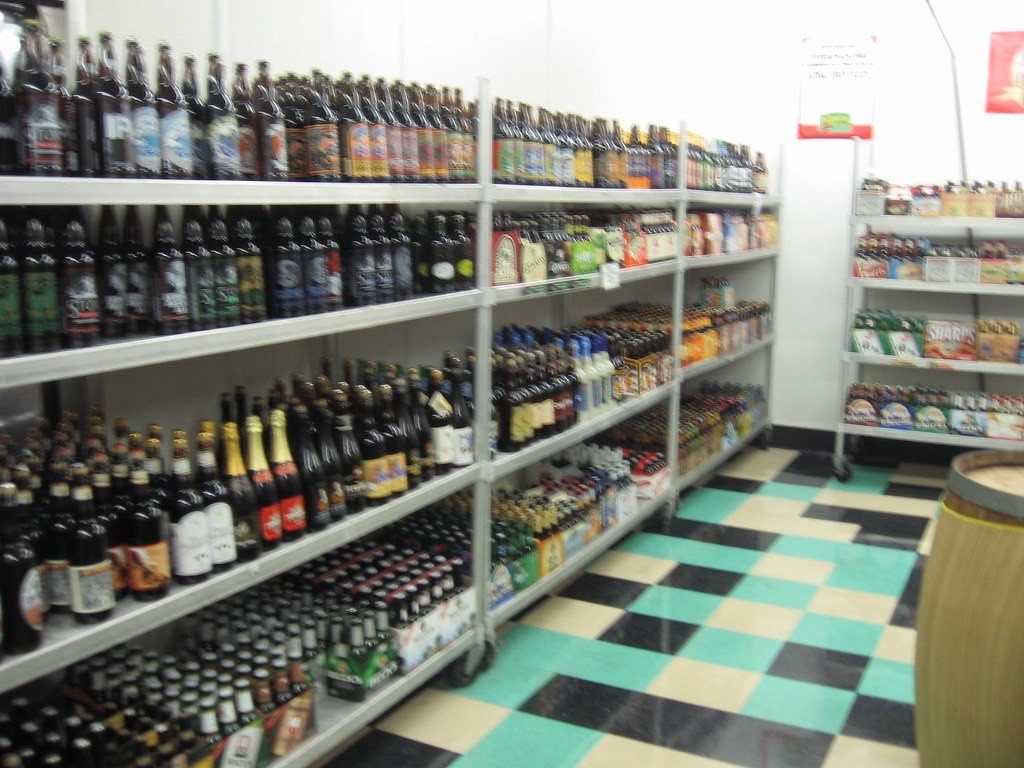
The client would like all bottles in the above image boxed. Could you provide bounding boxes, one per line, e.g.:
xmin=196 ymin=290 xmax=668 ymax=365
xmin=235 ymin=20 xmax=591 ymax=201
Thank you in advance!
xmin=853 ymin=305 xmax=1018 ymax=334
xmin=492 ymin=98 xmax=678 ymax=188
xmin=0 ymin=19 xmax=478 ymax=184
xmin=0 ymin=204 xmax=412 ymax=357
xmin=701 ymin=277 xmax=728 ymax=288
xmin=492 ymin=210 xmax=679 ymax=280
xmin=847 ymin=378 xmax=1024 ymax=414
xmin=467 ymin=302 xmax=773 ymax=608
xmin=411 ymin=209 xmax=478 ymax=299
xmin=685 ymin=132 xmax=768 ymax=195
xmin=0 ymin=351 xmax=474 ymax=768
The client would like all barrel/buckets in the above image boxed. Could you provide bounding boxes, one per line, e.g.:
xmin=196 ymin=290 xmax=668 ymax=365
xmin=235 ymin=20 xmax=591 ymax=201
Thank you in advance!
xmin=916 ymin=449 xmax=1024 ymax=768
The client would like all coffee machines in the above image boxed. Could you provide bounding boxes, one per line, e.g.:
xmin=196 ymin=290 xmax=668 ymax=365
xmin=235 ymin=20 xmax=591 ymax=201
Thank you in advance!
xmin=856 ymin=232 xmax=1024 ymax=262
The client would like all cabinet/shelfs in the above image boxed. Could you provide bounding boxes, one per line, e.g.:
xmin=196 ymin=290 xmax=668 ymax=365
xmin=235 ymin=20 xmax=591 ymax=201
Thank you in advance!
xmin=0 ymin=0 xmax=786 ymax=767
xmin=833 ymin=136 xmax=1023 ymax=482
